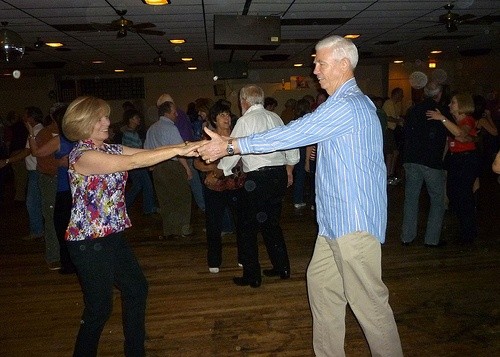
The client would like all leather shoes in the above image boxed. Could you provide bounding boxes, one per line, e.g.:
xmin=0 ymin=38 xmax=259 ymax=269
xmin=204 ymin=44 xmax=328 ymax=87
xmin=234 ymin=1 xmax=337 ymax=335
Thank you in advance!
xmin=263 ymin=268 xmax=290 ymax=280
xmin=164 ymin=231 xmax=201 ymax=241
xmin=233 ymin=277 xmax=262 ymax=288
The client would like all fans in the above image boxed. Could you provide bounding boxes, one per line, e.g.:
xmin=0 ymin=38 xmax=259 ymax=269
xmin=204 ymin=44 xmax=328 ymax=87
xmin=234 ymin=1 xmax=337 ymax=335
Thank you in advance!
xmin=409 ymin=2 xmax=500 ymax=35
xmin=21 ymin=37 xmax=71 ymax=53
xmin=51 ymin=10 xmax=166 ymax=39
xmin=130 ymin=50 xmax=187 ymax=68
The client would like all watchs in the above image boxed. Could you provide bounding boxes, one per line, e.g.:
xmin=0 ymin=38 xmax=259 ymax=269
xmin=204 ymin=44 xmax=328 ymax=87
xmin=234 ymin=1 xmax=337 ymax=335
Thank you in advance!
xmin=226 ymin=140 xmax=235 ymax=156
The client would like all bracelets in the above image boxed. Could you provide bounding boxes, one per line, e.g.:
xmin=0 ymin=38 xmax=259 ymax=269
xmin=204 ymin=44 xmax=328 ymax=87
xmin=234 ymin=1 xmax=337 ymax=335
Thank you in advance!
xmin=443 ymin=119 xmax=446 ymax=123
xmin=185 ymin=141 xmax=189 ymax=146
xmin=29 ymin=135 xmax=36 ymax=140
xmin=5 ymin=159 xmax=10 ymax=164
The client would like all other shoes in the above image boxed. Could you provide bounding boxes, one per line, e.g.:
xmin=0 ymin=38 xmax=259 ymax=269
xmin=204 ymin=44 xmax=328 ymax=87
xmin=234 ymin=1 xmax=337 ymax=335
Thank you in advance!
xmin=295 ymin=202 xmax=306 ymax=208
xmin=144 ymin=212 xmax=159 ymax=216
xmin=209 ymin=268 xmax=220 ymax=273
xmin=387 ymin=175 xmax=401 ymax=185
xmin=445 ymin=239 xmax=473 ymax=249
xmin=202 ymin=228 xmax=233 ymax=236
xmin=311 ymin=206 xmax=314 ymax=210
xmin=15 ymin=200 xmax=26 ymax=207
xmin=48 ymin=263 xmax=61 ymax=270
xmin=238 ymin=263 xmax=243 ymax=267
xmin=426 ymin=239 xmax=447 ymax=248
xmin=402 ymin=237 xmax=416 ymax=246
xmin=22 ymin=233 xmax=42 ymax=240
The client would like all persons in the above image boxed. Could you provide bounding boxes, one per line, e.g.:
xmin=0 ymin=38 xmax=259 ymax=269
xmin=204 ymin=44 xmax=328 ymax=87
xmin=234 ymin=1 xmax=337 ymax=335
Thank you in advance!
xmin=0 ymin=102 xmax=78 ymax=273
xmin=195 ymin=35 xmax=404 ymax=357
xmin=101 ymin=80 xmax=500 ymax=289
xmin=62 ymin=96 xmax=212 ymax=357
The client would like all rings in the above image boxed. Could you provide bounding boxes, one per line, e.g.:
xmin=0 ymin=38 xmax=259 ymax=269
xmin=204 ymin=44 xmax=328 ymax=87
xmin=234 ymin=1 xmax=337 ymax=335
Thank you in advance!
xmin=207 ymin=159 xmax=212 ymax=163
